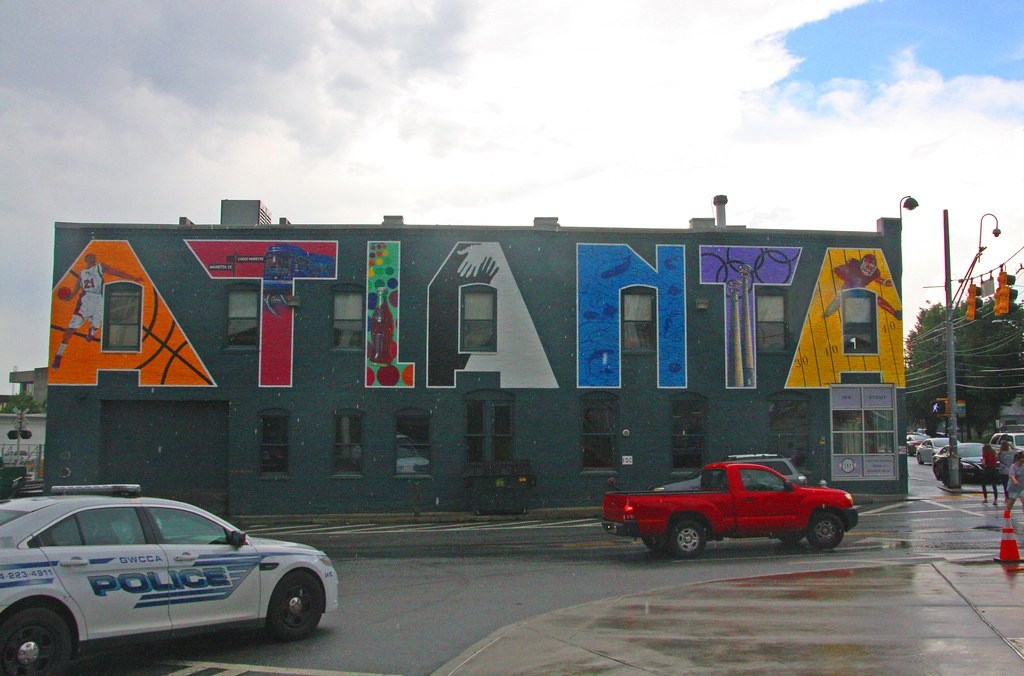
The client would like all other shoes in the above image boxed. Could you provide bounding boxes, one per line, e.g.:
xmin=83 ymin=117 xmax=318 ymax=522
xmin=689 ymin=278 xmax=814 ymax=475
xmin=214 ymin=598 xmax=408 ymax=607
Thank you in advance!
xmin=983 ymin=501 xmax=988 ymax=503
xmin=993 ymin=502 xmax=997 ymax=505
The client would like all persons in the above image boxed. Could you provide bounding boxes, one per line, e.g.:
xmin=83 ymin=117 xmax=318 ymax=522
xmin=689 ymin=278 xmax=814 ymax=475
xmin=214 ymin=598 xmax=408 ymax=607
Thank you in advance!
xmin=1006 ymin=451 xmax=1024 ymax=512
xmin=998 ymin=441 xmax=1021 ymax=502
xmin=51 ymin=254 xmax=143 ymax=369
xmin=981 ymin=444 xmax=998 ymax=505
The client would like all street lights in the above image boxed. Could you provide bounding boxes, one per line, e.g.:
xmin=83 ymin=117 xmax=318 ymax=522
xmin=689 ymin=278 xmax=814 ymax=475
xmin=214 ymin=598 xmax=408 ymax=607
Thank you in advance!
xmin=945 ymin=212 xmax=1002 ymax=488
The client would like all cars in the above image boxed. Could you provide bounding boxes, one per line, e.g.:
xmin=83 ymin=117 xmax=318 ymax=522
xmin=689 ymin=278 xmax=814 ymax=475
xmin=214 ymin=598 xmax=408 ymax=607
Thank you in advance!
xmin=0 ymin=482 xmax=339 ymax=676
xmin=932 ymin=442 xmax=986 ymax=484
xmin=916 ymin=437 xmax=961 ymax=465
xmin=906 ymin=434 xmax=932 ymax=456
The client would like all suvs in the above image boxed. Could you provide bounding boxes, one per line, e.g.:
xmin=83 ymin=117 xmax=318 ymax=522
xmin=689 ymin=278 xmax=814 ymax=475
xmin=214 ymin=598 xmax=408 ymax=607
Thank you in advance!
xmin=988 ymin=432 xmax=1024 ymax=470
xmin=648 ymin=451 xmax=807 ymax=513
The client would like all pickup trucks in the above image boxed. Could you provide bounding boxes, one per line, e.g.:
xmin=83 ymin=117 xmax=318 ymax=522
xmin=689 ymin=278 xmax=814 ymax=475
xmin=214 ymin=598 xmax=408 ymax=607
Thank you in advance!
xmin=600 ymin=460 xmax=859 ymax=560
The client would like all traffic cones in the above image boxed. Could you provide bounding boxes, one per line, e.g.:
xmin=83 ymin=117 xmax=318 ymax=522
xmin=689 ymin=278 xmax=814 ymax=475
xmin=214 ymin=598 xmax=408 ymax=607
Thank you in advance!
xmin=993 ymin=507 xmax=1024 ymax=563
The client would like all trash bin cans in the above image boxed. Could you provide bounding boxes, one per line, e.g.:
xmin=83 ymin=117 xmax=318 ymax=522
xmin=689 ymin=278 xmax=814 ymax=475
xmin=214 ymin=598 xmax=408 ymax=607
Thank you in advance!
xmin=466 ymin=461 xmax=538 ymax=516
xmin=942 ymin=456 xmax=962 ymax=488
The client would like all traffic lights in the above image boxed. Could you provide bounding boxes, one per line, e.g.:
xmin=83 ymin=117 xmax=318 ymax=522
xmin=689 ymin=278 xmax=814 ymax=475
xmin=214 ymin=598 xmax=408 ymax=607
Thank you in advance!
xmin=931 ymin=398 xmax=949 ymax=418
xmin=993 ymin=273 xmax=1019 ymax=315
xmin=965 ymin=284 xmax=983 ymax=321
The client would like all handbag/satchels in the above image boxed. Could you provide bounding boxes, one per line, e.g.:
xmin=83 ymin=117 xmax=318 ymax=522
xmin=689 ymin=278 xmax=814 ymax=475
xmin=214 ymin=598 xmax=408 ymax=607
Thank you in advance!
xmin=986 ymin=463 xmax=992 ymax=471
